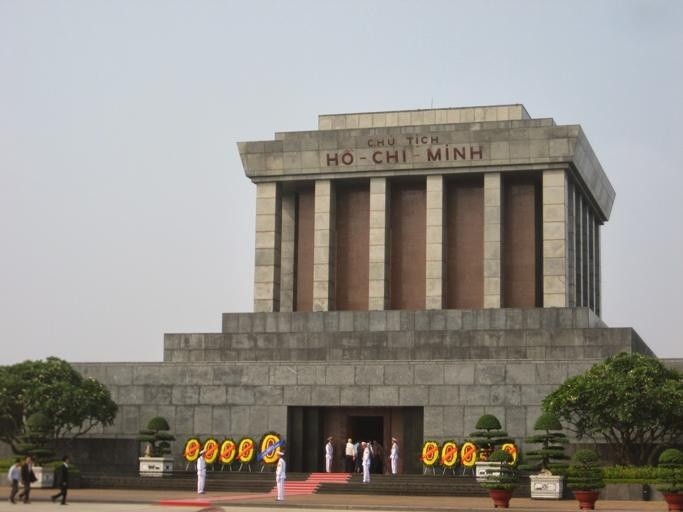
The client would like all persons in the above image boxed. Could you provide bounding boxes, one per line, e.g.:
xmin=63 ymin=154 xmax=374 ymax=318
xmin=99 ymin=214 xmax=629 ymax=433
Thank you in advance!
xmin=18 ymin=456 xmax=37 ymax=503
xmin=6 ymin=457 xmax=21 ymax=503
xmin=275 ymin=450 xmax=286 ymax=501
xmin=195 ymin=449 xmax=205 ymax=494
xmin=361 ymin=441 xmax=370 ymax=483
xmin=389 ymin=436 xmax=398 ymax=474
xmin=344 ymin=437 xmax=384 ymax=474
xmin=325 ymin=436 xmax=333 ymax=473
xmin=51 ymin=455 xmax=69 ymax=505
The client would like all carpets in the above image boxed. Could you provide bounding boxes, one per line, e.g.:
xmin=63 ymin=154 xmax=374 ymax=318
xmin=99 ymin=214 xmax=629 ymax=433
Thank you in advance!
xmin=150 ymin=472 xmax=353 ymax=506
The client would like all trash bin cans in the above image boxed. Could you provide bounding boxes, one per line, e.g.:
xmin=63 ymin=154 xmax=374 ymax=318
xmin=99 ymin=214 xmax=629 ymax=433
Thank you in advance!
xmin=643 ymin=484 xmax=650 ymax=501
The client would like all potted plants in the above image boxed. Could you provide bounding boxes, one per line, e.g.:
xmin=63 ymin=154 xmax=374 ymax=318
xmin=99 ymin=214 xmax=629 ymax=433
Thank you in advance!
xmin=14 ymin=411 xmax=56 ymax=488
xmin=134 ymin=417 xmax=177 ymax=478
xmin=470 ymin=413 xmax=607 ymax=511
xmin=653 ymin=448 xmax=683 ymax=512
